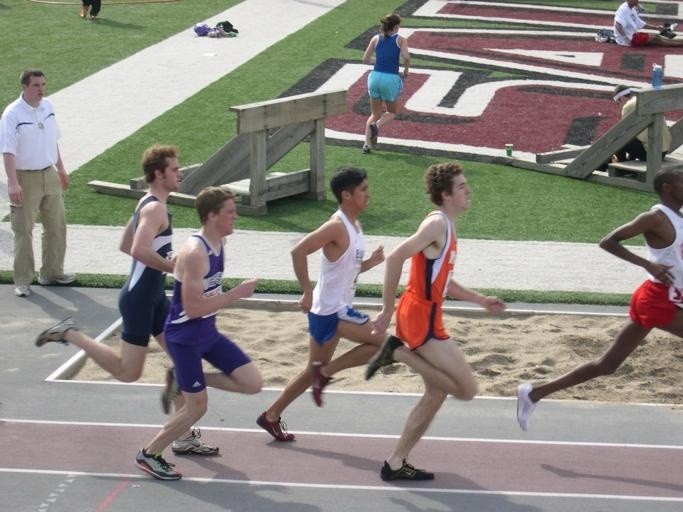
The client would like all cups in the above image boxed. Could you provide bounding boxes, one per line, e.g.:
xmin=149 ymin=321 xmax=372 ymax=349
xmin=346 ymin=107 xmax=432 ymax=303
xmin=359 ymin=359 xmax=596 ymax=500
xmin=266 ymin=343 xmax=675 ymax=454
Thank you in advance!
xmin=504 ymin=143 xmax=513 ymax=157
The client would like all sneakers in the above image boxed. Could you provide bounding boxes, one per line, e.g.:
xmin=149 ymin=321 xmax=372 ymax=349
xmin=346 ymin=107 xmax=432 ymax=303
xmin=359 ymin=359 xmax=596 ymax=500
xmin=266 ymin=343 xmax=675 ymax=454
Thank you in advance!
xmin=37 ymin=272 xmax=76 ymax=284
xmin=160 ymin=366 xmax=181 ymax=415
xmin=379 ymin=459 xmax=435 ymax=482
xmin=171 ymin=437 xmax=219 ymax=455
xmin=515 ymin=382 xmax=535 ymax=430
xmin=80 ymin=5 xmax=96 ymax=19
xmin=15 ymin=284 xmax=31 ymax=296
xmin=362 ymin=121 xmax=378 ymax=154
xmin=134 ymin=447 xmax=182 ymax=481
xmin=35 ymin=315 xmax=79 ymax=347
xmin=257 ymin=411 xmax=295 ymax=442
xmin=311 ymin=361 xmax=332 ymax=406
xmin=362 ymin=333 xmax=403 ymax=380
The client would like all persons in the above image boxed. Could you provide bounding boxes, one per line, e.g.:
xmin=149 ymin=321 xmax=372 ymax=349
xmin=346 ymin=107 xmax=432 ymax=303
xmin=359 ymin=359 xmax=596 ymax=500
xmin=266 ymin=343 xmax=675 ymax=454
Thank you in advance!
xmin=80 ymin=0 xmax=101 ymax=19
xmin=33 ymin=145 xmax=220 ymax=457
xmin=0 ymin=69 xmax=76 ymax=297
xmin=256 ymin=166 xmax=390 ymax=441
xmin=614 ymin=0 xmax=683 ymax=47
xmin=135 ymin=186 xmax=262 ymax=480
xmin=515 ymin=163 xmax=683 ymax=433
xmin=366 ymin=162 xmax=506 ymax=481
xmin=361 ymin=13 xmax=410 ymax=154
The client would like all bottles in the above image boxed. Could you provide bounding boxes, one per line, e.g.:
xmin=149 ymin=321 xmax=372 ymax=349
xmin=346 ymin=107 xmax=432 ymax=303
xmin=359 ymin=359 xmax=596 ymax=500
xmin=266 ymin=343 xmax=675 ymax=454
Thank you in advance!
xmin=650 ymin=63 xmax=663 ymax=88
